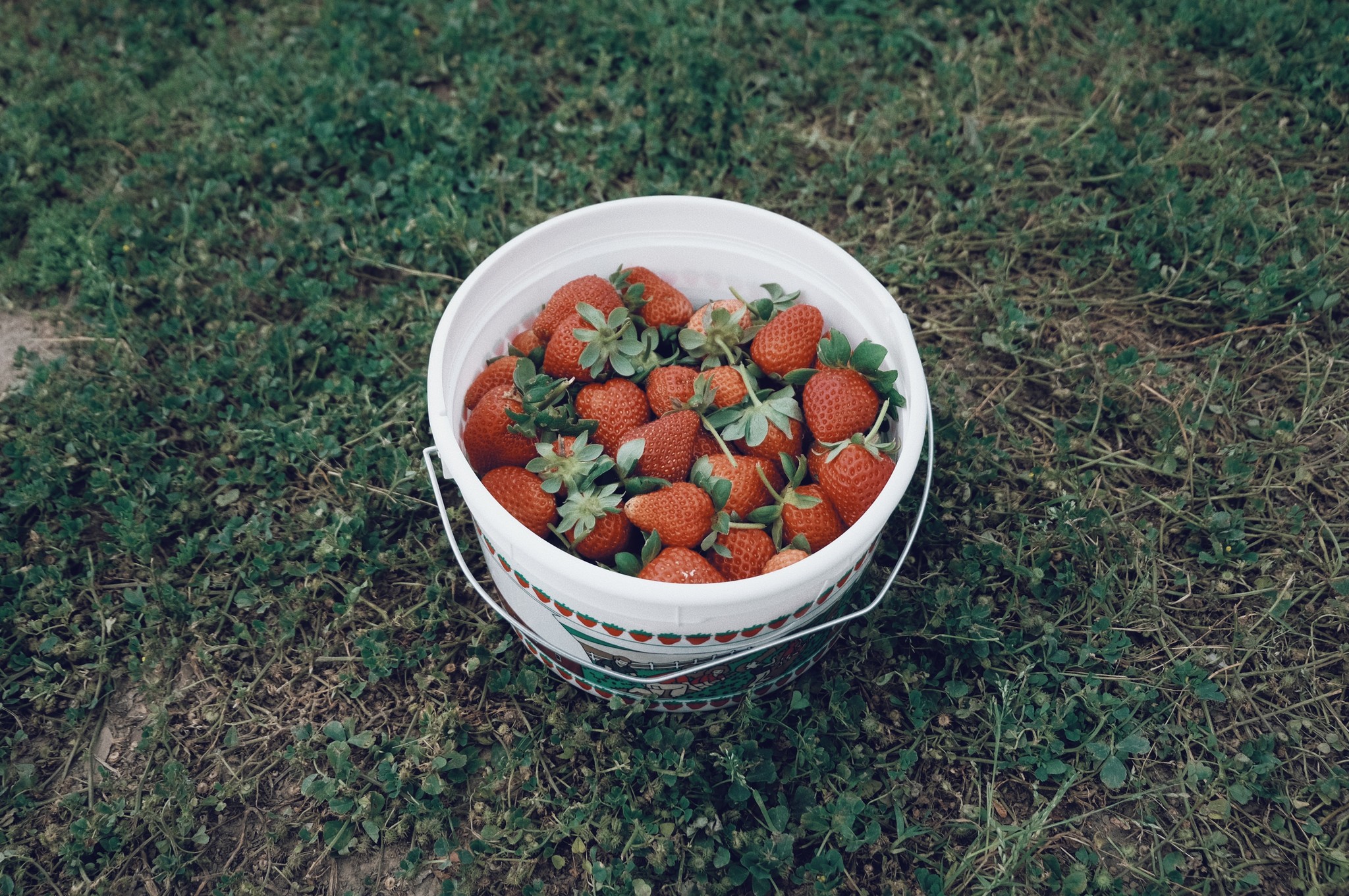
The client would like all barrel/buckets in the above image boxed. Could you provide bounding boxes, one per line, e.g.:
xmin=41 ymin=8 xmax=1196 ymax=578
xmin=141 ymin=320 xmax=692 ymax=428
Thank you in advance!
xmin=421 ymin=195 xmax=934 ymax=712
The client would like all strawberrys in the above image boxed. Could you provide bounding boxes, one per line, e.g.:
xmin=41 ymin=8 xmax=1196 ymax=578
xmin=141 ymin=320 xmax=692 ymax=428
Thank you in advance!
xmin=461 ymin=267 xmax=906 ymax=584
